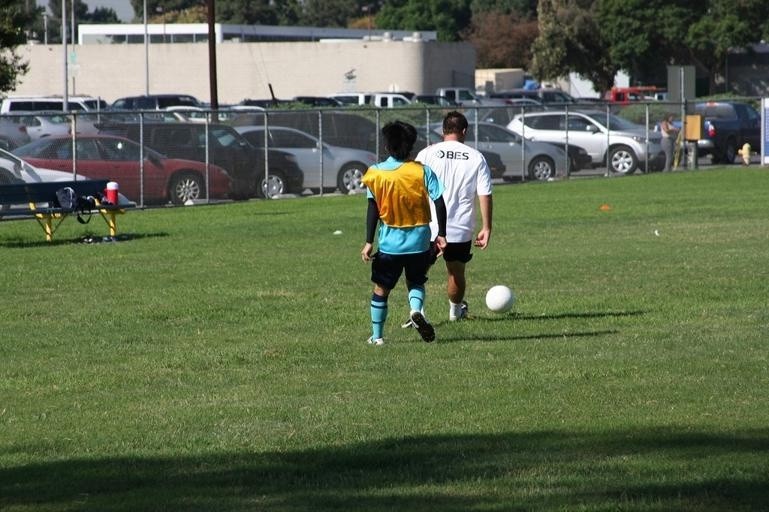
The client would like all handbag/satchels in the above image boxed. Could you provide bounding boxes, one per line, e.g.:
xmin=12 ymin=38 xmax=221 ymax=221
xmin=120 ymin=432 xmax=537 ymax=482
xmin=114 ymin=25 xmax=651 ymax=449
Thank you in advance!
xmin=50 ymin=185 xmax=97 ymax=225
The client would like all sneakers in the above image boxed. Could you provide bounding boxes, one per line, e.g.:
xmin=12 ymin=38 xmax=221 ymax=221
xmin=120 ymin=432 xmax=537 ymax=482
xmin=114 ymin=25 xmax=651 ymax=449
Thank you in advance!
xmin=401 ymin=308 xmax=439 ymax=344
xmin=450 ymin=299 xmax=471 ymax=323
xmin=364 ymin=333 xmax=388 ymax=347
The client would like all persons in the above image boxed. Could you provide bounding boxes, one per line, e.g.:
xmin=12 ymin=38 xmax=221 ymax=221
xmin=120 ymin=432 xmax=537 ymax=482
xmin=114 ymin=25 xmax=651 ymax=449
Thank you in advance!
xmin=399 ymin=109 xmax=494 ymax=330
xmin=659 ymin=112 xmax=681 ymax=173
xmin=358 ymin=118 xmax=451 ymax=345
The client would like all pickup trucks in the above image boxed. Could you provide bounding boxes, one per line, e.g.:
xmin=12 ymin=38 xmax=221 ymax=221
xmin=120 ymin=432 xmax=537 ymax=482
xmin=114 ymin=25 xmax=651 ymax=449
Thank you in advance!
xmin=651 ymin=95 xmax=760 ymax=164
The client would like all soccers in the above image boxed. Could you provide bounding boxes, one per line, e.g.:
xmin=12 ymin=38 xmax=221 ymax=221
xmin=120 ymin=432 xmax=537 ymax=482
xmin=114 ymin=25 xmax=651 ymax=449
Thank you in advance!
xmin=485 ymin=285 xmax=514 ymax=313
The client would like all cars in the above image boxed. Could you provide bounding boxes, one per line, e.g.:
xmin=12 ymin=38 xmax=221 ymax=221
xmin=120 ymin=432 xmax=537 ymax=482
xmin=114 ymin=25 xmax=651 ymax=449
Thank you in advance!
xmin=505 ymin=111 xmax=668 ymax=176
xmin=413 ymin=126 xmax=507 ymax=179
xmin=7 ymin=134 xmax=235 ymax=207
xmin=200 ymin=124 xmax=378 ymax=195
xmin=0 ymin=147 xmax=91 ymax=212
xmin=0 ymin=86 xmax=659 ymax=150
xmin=424 ymin=121 xmax=571 ymax=182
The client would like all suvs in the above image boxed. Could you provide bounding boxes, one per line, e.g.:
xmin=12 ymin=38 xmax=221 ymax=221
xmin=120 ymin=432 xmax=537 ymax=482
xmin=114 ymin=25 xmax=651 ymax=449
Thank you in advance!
xmin=93 ymin=120 xmax=304 ymax=200
xmin=235 ymin=111 xmax=431 ymax=176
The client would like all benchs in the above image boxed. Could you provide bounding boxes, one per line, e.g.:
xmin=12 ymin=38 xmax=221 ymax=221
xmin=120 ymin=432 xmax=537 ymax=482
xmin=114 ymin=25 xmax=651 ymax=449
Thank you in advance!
xmin=0 ymin=178 xmax=138 ymax=242
